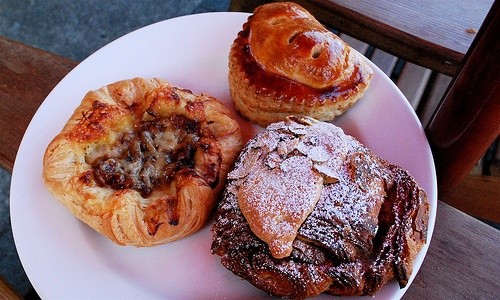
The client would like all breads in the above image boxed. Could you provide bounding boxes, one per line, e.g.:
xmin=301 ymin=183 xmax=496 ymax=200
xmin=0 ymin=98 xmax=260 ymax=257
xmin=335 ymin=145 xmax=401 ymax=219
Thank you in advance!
xmin=208 ymin=114 xmax=431 ymax=300
xmin=228 ymin=2 xmax=374 ymax=128
xmin=42 ymin=76 xmax=243 ymax=249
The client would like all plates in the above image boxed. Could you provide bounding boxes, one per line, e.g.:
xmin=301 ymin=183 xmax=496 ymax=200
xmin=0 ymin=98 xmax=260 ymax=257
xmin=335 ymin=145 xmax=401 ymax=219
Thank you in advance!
xmin=9 ymin=11 xmax=438 ymax=299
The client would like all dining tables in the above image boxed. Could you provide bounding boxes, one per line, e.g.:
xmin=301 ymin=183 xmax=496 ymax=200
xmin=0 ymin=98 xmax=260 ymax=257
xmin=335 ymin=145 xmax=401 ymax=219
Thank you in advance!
xmin=1 ymin=37 xmax=500 ymax=300
